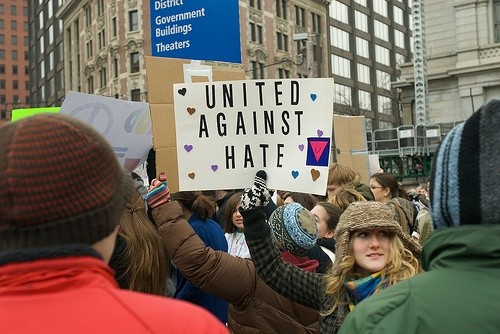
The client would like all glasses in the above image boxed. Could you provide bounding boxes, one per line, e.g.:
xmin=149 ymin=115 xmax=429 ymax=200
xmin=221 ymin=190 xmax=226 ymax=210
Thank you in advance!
xmin=373 ymin=185 xmax=381 ymax=188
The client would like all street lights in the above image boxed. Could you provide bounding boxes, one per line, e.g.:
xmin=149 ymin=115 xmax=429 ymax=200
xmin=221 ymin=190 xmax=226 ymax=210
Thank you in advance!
xmin=245 ymin=47 xmax=307 ymax=72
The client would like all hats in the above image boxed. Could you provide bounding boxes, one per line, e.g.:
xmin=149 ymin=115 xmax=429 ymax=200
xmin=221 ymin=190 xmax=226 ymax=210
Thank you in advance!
xmin=429 ymin=99 xmax=499 ymax=232
xmin=334 ymin=200 xmax=401 ymax=237
xmin=269 ymin=202 xmax=319 ymax=256
xmin=1 ymin=113 xmax=133 ymax=246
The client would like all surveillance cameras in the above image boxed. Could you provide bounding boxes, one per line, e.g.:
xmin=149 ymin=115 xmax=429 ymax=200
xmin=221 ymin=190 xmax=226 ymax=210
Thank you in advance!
xmin=293 ymin=32 xmax=307 ymax=41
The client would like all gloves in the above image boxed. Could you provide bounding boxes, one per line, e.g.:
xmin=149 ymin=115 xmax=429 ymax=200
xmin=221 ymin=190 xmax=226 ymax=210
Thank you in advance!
xmin=240 ymin=170 xmax=273 ymax=209
xmin=145 ymin=173 xmax=170 ymax=207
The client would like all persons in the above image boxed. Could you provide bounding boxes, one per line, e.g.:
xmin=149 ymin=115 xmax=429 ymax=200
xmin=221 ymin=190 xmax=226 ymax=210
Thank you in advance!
xmin=239 ymin=170 xmax=421 ymax=334
xmin=0 ymin=110 xmax=233 ymax=333
xmin=336 ymin=97 xmax=500 ymax=334
xmin=93 ymin=160 xmax=433 ymax=326
